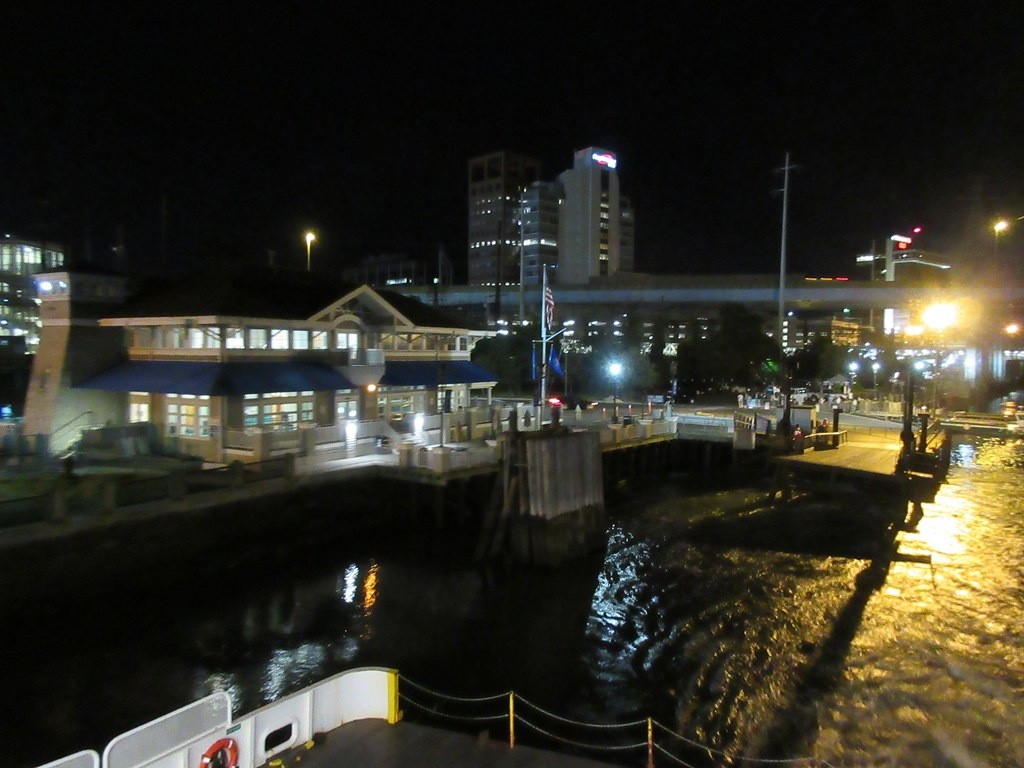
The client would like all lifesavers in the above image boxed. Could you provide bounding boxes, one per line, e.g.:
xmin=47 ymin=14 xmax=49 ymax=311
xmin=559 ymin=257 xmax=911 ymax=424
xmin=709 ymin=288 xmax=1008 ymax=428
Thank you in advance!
xmin=202 ymin=737 xmax=238 ymax=767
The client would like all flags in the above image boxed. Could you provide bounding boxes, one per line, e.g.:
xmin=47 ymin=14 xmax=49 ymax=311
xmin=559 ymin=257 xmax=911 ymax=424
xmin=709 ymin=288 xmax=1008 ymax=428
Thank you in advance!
xmin=532 ymin=343 xmax=536 ymax=381
xmin=545 ymin=270 xmax=554 ymax=330
xmin=549 ymin=343 xmax=565 ymax=377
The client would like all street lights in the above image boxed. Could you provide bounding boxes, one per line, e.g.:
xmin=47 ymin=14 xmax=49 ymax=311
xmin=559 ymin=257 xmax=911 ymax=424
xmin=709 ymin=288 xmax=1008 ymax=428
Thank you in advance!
xmin=610 ymin=361 xmax=624 ymax=426
xmin=305 ymin=232 xmax=315 ymax=273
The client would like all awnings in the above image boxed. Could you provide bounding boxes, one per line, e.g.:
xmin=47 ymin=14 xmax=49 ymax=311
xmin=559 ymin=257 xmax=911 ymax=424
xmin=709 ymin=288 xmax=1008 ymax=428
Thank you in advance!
xmin=70 ymin=359 xmax=360 ymax=397
xmin=377 ymin=360 xmax=499 ymax=387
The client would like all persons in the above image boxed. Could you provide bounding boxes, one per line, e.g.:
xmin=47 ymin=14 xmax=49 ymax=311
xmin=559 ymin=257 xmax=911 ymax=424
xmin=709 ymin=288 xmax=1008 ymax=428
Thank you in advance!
xmin=737 ymin=394 xmax=744 ymax=408
xmin=811 ymin=418 xmax=829 ymax=444
xmin=1 ymin=404 xmax=15 ymax=420
xmin=793 ymin=423 xmax=803 ymax=454
xmin=853 ymin=398 xmax=858 ymax=411
